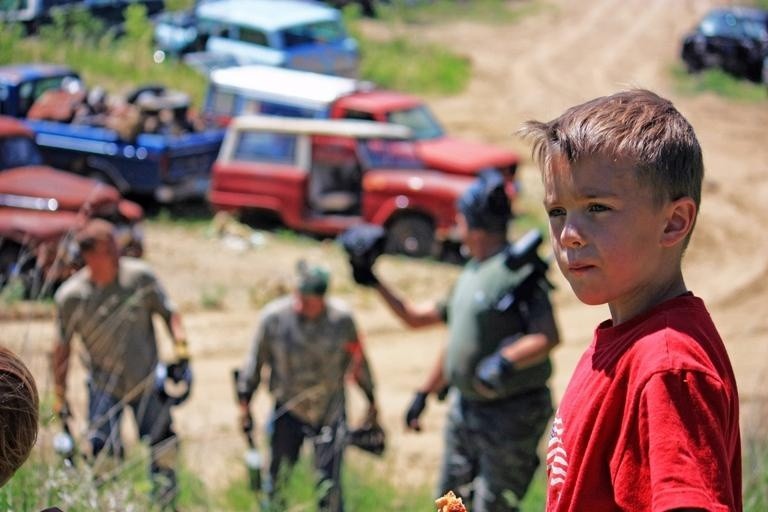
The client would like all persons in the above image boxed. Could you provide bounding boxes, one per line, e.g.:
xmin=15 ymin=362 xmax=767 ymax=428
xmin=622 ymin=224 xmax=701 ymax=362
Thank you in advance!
xmin=1 ymin=345 xmax=40 ymax=483
xmin=52 ymin=218 xmax=191 ymax=512
xmin=236 ymin=268 xmax=379 ymax=512
xmin=0 ymin=1 xmax=165 ymax=44
xmin=511 ymin=80 xmax=742 ymax=511
xmin=405 ymin=166 xmax=563 ymax=511
xmin=353 ymin=259 xmax=450 ymax=329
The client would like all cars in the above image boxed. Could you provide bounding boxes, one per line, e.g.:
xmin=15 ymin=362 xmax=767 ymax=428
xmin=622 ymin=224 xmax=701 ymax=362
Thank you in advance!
xmin=681 ymin=10 xmax=767 ymax=83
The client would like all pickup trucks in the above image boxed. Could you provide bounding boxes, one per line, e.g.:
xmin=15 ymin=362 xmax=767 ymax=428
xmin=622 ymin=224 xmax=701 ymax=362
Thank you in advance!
xmin=0 ymin=63 xmax=227 ymax=212
xmin=0 ymin=113 xmax=148 ymax=303
xmin=204 ymin=62 xmax=518 ymax=187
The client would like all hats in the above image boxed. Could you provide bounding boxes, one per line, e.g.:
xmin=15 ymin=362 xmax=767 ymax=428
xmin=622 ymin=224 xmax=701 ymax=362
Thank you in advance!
xmin=460 ymin=170 xmax=510 ymax=231
xmin=344 ymin=226 xmax=387 ymax=282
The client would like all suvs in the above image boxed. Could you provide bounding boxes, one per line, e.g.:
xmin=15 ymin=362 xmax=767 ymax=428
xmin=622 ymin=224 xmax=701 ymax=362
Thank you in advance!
xmin=153 ymin=0 xmax=364 ymax=76
xmin=206 ymin=112 xmax=520 ymax=258
